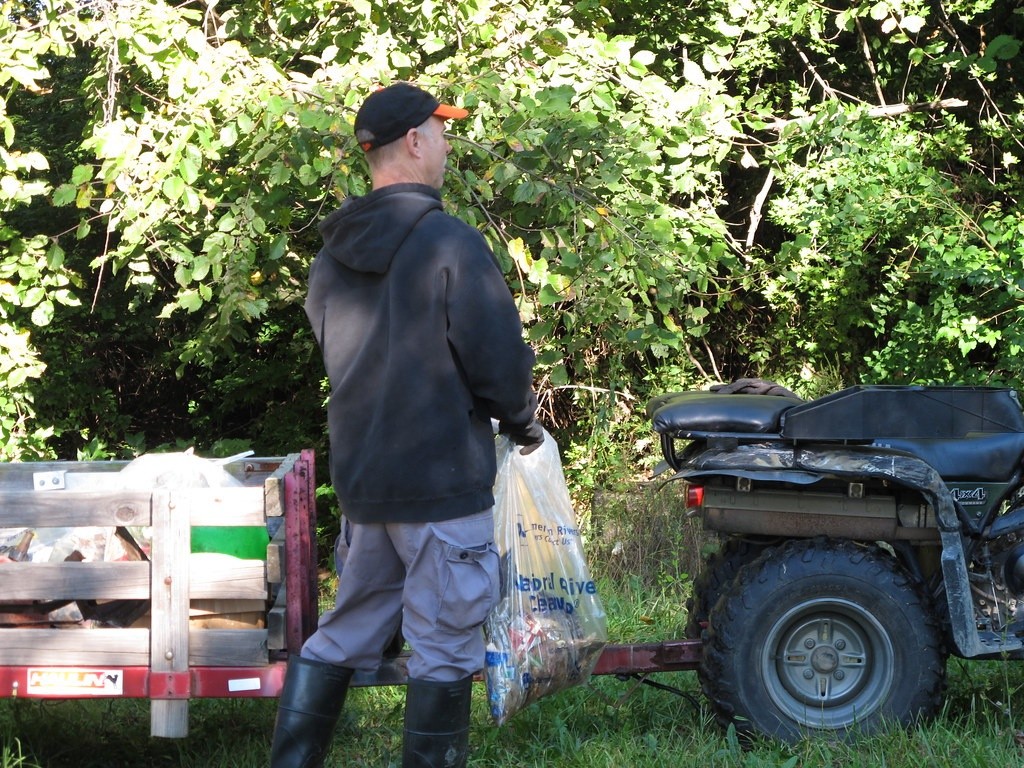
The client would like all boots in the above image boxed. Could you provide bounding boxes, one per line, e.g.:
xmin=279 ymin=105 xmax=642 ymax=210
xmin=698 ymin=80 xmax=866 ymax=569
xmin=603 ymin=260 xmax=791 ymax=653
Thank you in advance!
xmin=403 ymin=675 xmax=473 ymax=768
xmin=270 ymin=653 xmax=356 ymax=768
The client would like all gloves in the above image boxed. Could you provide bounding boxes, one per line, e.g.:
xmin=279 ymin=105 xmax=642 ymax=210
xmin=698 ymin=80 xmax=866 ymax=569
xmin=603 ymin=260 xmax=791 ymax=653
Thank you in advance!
xmin=498 ymin=419 xmax=544 ymax=456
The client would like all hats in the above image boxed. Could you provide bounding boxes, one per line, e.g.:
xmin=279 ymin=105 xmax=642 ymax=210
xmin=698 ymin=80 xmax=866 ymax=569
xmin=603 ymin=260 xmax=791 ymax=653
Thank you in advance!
xmin=354 ymin=84 xmax=469 ymax=152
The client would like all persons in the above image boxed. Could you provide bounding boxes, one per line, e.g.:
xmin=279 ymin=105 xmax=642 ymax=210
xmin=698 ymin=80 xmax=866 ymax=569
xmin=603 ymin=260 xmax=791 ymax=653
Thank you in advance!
xmin=267 ymin=82 xmax=545 ymax=768
